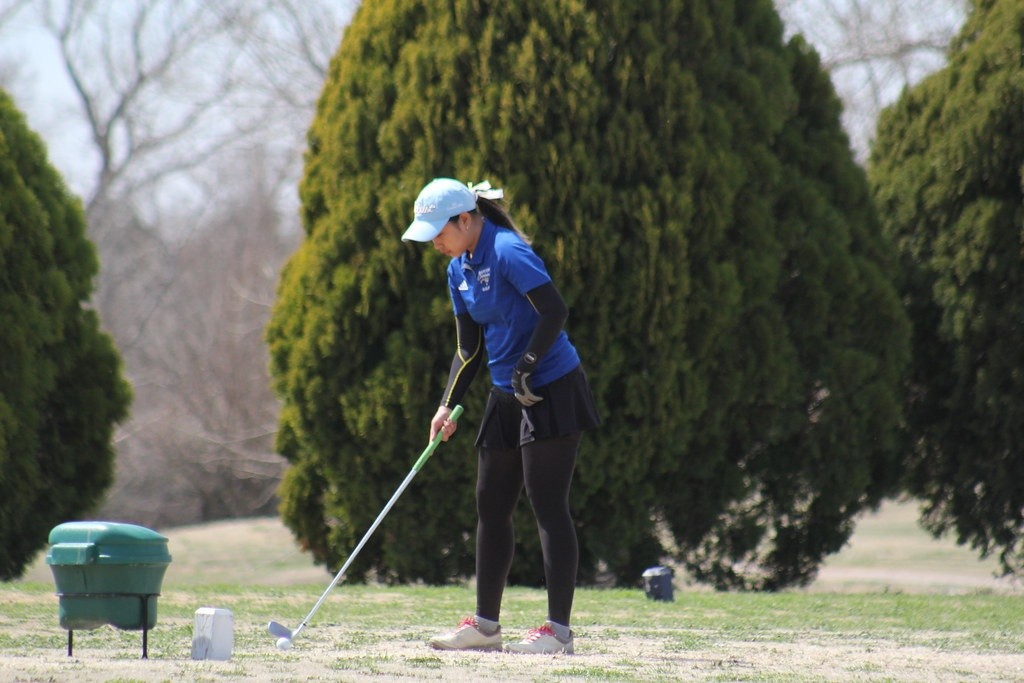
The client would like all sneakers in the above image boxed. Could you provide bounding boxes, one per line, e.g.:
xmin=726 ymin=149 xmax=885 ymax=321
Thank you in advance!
xmin=428 ymin=619 xmax=503 ymax=652
xmin=502 ymin=625 xmax=575 ymax=655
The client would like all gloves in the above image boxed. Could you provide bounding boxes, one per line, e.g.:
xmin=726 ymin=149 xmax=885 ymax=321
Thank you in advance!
xmin=511 ymin=368 xmax=543 ymax=406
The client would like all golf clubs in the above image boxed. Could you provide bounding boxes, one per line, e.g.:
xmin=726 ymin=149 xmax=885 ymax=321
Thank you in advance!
xmin=265 ymin=404 xmax=465 ymax=641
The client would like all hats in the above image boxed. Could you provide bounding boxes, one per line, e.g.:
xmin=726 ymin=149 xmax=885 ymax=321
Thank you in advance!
xmin=400 ymin=179 xmax=476 ymax=242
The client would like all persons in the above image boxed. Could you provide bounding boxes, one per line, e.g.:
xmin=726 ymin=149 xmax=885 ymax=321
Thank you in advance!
xmin=400 ymin=176 xmax=604 ymax=656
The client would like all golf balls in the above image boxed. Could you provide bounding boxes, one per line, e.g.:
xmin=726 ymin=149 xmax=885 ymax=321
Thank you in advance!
xmin=275 ymin=638 xmax=291 ymax=651
